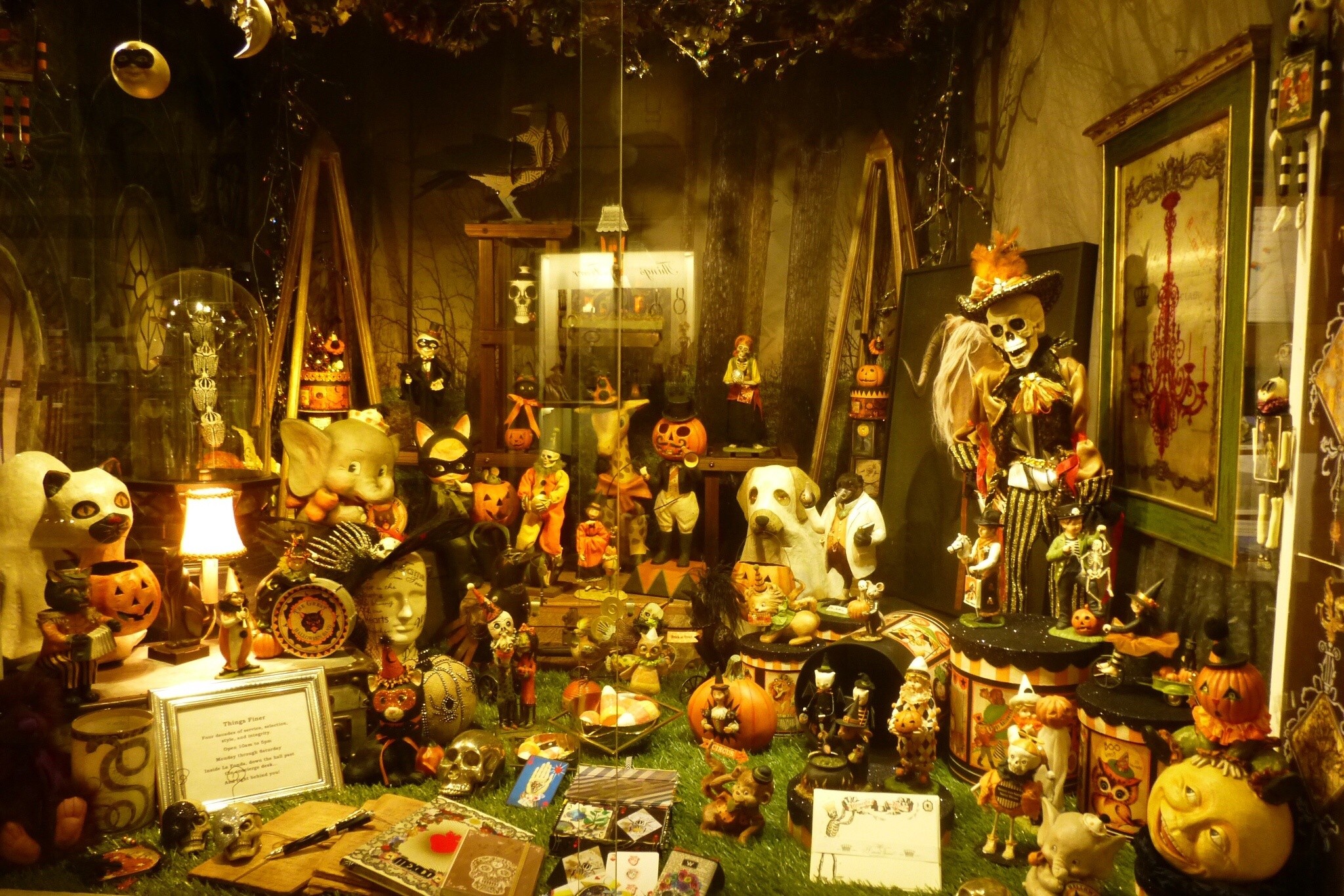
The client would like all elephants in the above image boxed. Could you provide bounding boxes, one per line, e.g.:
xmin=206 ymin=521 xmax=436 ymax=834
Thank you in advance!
xmin=278 ymin=408 xmax=408 ymax=564
xmin=1025 ymin=798 xmax=1127 ymax=896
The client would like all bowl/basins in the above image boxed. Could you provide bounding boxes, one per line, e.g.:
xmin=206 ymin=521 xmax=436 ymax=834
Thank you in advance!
xmin=518 ymin=732 xmax=578 ymax=773
xmin=568 ymin=692 xmax=660 ymax=752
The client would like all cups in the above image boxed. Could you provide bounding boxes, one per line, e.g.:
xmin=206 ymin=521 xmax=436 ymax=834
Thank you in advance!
xmin=70 ymin=709 xmax=156 ymax=833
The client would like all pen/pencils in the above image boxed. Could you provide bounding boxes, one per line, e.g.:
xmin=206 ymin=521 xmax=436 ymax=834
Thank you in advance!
xmin=263 ymin=809 xmax=375 ymax=860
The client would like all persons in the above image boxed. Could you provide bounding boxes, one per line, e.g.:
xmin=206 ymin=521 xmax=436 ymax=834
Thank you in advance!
xmin=799 ymin=471 xmax=888 ymax=601
xmin=700 ymin=666 xmax=742 ymax=743
xmin=350 ymin=547 xmax=540 ymax=788
xmin=800 ymin=646 xmax=836 ymax=750
xmin=887 ymin=655 xmax=942 ymax=789
xmin=515 ymin=426 xmax=571 ymax=577
xmin=400 ymin=321 xmax=451 ymax=424
xmin=211 ymin=560 xmax=265 ymax=678
xmin=837 ymin=671 xmax=878 ymax=764
xmin=961 ymin=507 xmax=1005 ymax=623
xmin=970 ymin=673 xmax=1073 ymax=860
xmin=574 ymin=395 xmax=708 ymax=595
xmin=721 ymin=334 xmax=769 ymax=450
xmin=926 ymin=231 xmax=1115 ymax=619
xmin=1044 ymin=504 xmax=1099 ymax=633
xmin=855 ymin=364 xmax=883 ymax=387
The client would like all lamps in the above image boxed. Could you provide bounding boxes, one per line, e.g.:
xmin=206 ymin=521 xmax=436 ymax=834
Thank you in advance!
xmin=178 ymin=488 xmax=248 ymax=646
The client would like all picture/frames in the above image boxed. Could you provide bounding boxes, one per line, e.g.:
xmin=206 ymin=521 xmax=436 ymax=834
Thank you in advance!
xmin=1083 ymin=24 xmax=1272 ymax=570
xmin=147 ymin=666 xmax=345 ymax=823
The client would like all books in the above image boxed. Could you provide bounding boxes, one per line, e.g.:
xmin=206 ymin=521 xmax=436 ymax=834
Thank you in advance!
xmin=188 ymin=790 xmax=548 ymax=896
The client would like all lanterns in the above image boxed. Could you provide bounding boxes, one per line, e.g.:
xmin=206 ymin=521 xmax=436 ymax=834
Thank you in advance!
xmin=79 ymin=560 xmax=162 ymax=638
xmin=473 ymin=465 xmax=519 ymax=529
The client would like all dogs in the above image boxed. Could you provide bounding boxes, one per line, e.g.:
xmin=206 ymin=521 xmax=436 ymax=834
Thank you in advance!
xmin=736 ymin=464 xmax=844 ymax=605
